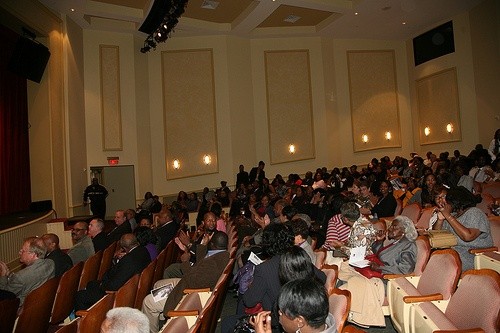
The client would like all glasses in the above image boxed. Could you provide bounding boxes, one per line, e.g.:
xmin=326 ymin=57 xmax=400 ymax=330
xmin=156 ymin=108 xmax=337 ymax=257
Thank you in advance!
xmin=388 ymin=224 xmax=402 ymax=229
xmin=139 ymin=223 xmax=150 ymax=226
xmin=71 ymin=228 xmax=85 ymax=232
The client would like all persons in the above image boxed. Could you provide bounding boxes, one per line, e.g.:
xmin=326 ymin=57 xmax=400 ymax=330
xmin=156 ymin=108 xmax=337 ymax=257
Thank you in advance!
xmin=223 ymin=172 xmax=336 ymax=333
xmin=488 ymin=129 xmax=500 ymax=160
xmin=236 ymin=161 xmax=265 ymax=188
xmin=88 ymin=219 xmax=108 ymax=252
xmin=83 ymin=178 xmax=109 ymax=219
xmin=305 ymin=155 xmax=418 ymax=328
xmin=393 ymin=144 xmax=500 ymax=288
xmin=100 ymin=181 xmax=231 ymax=333
xmin=66 ymin=223 xmax=95 ymax=265
xmin=0 ymin=236 xmax=56 ymax=315
xmin=41 ymin=234 xmax=73 ymax=279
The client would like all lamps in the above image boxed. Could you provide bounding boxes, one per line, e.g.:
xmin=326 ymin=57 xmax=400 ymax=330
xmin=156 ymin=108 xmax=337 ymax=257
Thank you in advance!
xmin=447 ymin=123 xmax=454 ymax=134
xmin=140 ymin=0 xmax=187 ymax=53
xmin=386 ymin=130 xmax=392 ymax=141
xmin=425 ymin=126 xmax=430 ymax=137
xmin=289 ymin=144 xmax=294 ymax=155
xmin=205 ymin=154 xmax=209 ymax=165
xmin=364 ymin=135 xmax=368 ymax=144
xmin=174 ymin=160 xmax=178 ymax=170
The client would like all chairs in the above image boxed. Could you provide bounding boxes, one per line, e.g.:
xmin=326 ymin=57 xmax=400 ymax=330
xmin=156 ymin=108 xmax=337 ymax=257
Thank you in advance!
xmin=0 ymin=182 xmax=500 ymax=333
